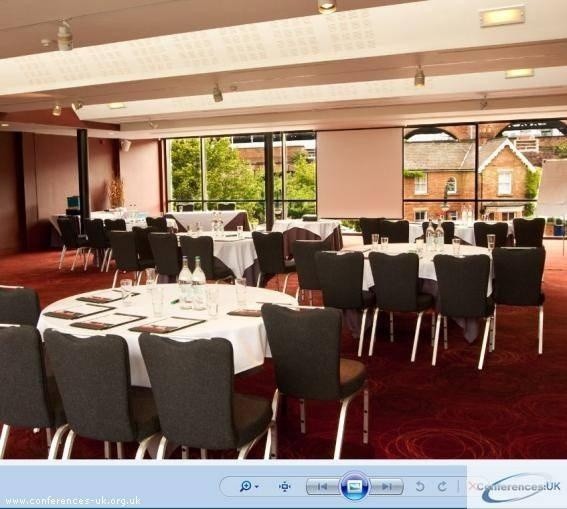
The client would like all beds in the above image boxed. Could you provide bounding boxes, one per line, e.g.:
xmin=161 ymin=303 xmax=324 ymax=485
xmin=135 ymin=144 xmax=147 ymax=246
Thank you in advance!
xmin=269 ymin=219 xmax=342 ymax=260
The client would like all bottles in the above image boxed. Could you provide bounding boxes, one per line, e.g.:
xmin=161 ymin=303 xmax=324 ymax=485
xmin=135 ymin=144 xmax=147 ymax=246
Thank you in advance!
xmin=462 ymin=203 xmax=468 ymax=224
xmin=191 ymin=256 xmax=208 ymax=311
xmin=211 ymin=212 xmax=217 ymax=237
xmin=426 ymin=218 xmax=434 ymax=252
xmin=468 ymin=203 xmax=473 ymax=224
xmin=178 ymin=255 xmax=193 ymax=309
xmin=434 ymin=218 xmax=445 ymax=252
xmin=218 ymin=212 xmax=225 ymax=237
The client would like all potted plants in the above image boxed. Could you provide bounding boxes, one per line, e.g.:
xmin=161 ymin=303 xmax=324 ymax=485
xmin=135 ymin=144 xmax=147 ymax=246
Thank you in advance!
xmin=543 ymin=217 xmax=565 ymax=237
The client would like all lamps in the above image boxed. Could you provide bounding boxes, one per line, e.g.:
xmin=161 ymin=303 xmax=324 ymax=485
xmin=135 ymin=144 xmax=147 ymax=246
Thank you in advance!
xmin=120 ymin=139 xmax=132 ymax=152
xmin=413 ymin=66 xmax=425 ymax=87
xmin=315 ymin=1 xmax=337 ymax=15
xmin=213 ymin=82 xmax=223 ymax=102
xmin=73 ymin=100 xmax=83 ymax=110
xmin=149 ymin=121 xmax=157 ymax=130
xmin=478 ymin=4 xmax=524 ymax=29
xmin=505 ymin=70 xmax=534 ymax=79
xmin=53 ymin=103 xmax=62 ymax=115
xmin=56 ymin=20 xmax=74 ymax=53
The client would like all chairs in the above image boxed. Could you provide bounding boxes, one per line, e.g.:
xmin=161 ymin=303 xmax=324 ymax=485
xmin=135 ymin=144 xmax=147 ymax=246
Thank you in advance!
xmin=49 ymin=202 xmax=237 ymax=290
xmin=291 ymin=216 xmax=547 ymax=370
xmin=251 ymin=231 xmax=297 ymax=294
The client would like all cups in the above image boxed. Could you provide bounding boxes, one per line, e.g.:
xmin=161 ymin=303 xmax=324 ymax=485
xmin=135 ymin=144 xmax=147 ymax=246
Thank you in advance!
xmin=451 ymin=239 xmax=461 ymax=257
xmin=120 ymin=279 xmax=133 ymax=306
xmin=151 ymin=285 xmax=166 ymax=319
xmin=187 ymin=224 xmax=192 ymax=236
xmin=249 ymin=223 xmax=257 ymax=236
xmin=195 ymin=222 xmax=200 ymax=232
xmin=417 ymin=239 xmax=424 ymax=258
xmin=372 ymin=233 xmax=379 ymax=252
xmin=196 ymin=226 xmax=202 ymax=236
xmin=237 ymin=226 xmax=243 ymax=239
xmin=381 ymin=237 xmax=389 ymax=251
xmin=487 ymin=233 xmax=497 ymax=251
xmin=235 ymin=277 xmax=247 ymax=308
xmin=178 ymin=206 xmax=184 ymax=213
xmin=145 ymin=267 xmax=157 ymax=294
